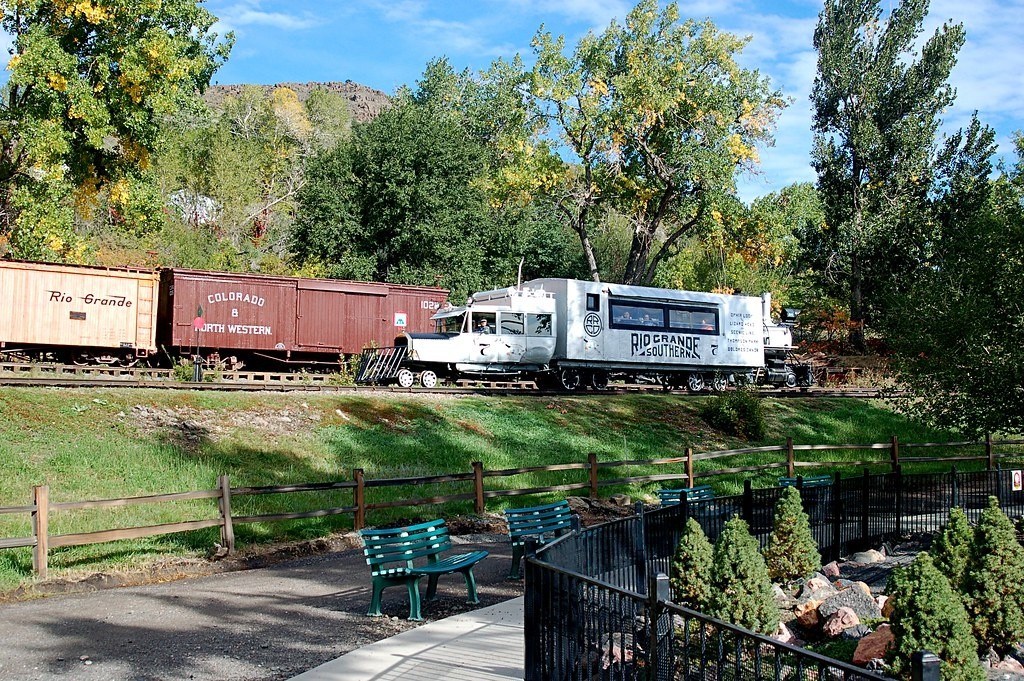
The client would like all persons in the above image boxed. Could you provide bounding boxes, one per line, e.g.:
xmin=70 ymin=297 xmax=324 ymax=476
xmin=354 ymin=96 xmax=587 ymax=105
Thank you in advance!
xmin=699 ymin=319 xmax=713 ymax=330
xmin=619 ymin=311 xmax=630 ymax=321
xmin=643 ymin=313 xmax=651 ymax=321
xmin=476 ymin=317 xmax=490 ymax=334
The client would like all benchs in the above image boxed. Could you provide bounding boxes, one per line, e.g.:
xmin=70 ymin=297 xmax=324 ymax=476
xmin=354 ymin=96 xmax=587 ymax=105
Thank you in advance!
xmin=358 ymin=519 xmax=489 ymax=622
xmin=657 ymin=485 xmax=735 ymax=546
xmin=777 ymin=475 xmax=847 ymax=523
xmin=503 ymin=499 xmax=572 ymax=579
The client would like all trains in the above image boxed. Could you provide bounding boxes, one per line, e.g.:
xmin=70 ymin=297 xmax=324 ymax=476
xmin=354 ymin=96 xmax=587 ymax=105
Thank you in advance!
xmin=352 ymin=276 xmax=819 ymax=396
xmin=1 ymin=258 xmax=452 ymax=370
